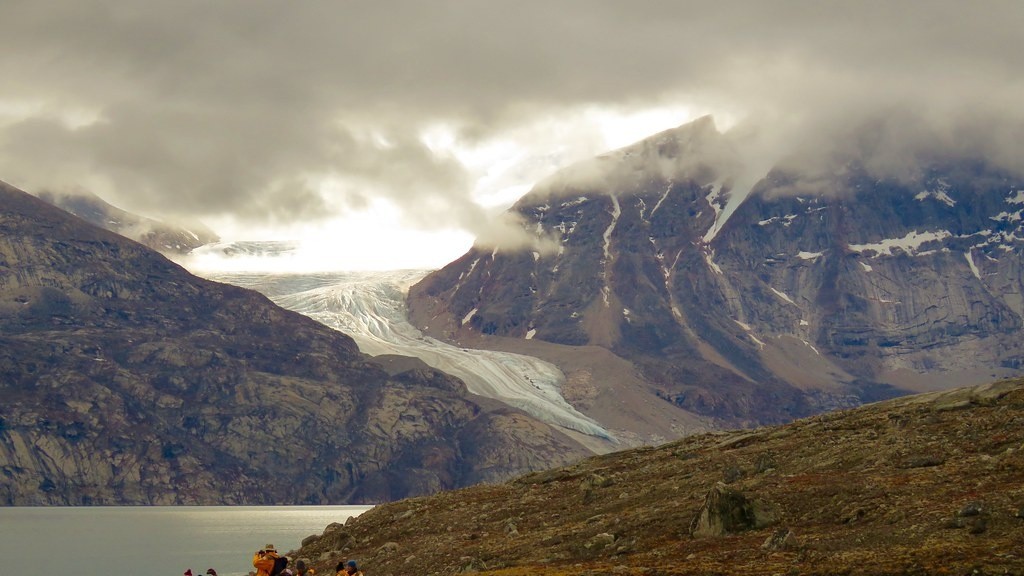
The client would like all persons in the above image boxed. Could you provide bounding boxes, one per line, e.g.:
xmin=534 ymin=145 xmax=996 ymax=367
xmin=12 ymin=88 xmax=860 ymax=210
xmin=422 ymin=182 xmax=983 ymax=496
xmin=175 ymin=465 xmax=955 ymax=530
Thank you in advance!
xmin=254 ymin=544 xmax=363 ymax=576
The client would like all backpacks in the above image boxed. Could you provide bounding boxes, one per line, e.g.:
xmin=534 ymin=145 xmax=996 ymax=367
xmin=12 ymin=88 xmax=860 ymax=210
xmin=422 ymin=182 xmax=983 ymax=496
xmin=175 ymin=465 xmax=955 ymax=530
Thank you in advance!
xmin=269 ymin=554 xmax=288 ymax=576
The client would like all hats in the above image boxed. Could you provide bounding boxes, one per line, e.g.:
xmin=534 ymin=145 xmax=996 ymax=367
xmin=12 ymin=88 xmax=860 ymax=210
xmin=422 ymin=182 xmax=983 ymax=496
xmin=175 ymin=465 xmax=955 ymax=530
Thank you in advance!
xmin=347 ymin=560 xmax=356 ymax=566
xmin=265 ymin=543 xmax=274 ymax=550
